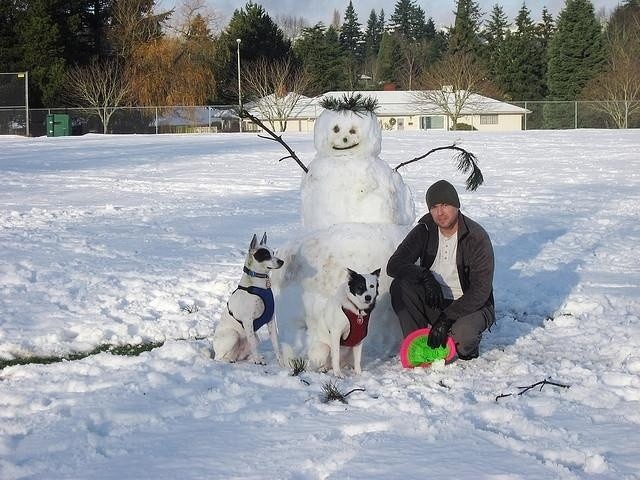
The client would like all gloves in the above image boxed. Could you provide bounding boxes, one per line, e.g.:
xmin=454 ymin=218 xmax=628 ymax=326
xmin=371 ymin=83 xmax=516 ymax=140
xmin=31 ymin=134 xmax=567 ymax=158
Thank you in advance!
xmin=418 ymin=268 xmax=445 ymax=309
xmin=427 ymin=311 xmax=457 ymax=350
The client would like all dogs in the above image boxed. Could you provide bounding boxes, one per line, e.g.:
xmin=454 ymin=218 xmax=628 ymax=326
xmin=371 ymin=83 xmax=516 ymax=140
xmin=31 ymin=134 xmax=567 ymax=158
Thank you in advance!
xmin=210 ymin=231 xmax=284 ymax=366
xmin=308 ymin=267 xmax=383 ymax=378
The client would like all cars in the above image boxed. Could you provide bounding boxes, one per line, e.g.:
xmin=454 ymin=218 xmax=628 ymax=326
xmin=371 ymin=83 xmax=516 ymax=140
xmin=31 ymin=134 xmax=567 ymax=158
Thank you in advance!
xmin=9 ymin=115 xmax=31 ymax=132
xmin=72 ymin=117 xmax=89 ymax=134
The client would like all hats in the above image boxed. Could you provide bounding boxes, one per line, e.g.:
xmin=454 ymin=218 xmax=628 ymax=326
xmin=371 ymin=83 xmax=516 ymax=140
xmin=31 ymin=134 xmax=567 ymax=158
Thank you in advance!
xmin=426 ymin=177 xmax=460 ymax=208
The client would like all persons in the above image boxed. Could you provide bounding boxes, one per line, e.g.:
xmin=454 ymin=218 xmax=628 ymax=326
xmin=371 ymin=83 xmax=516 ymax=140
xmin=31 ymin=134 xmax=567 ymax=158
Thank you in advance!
xmin=385 ymin=177 xmax=497 ymax=362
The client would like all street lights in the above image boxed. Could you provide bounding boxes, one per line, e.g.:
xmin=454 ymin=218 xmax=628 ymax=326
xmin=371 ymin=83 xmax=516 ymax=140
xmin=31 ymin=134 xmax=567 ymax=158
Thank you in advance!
xmin=18 ymin=71 xmax=30 ymax=135
xmin=234 ymin=38 xmax=244 ymax=133
